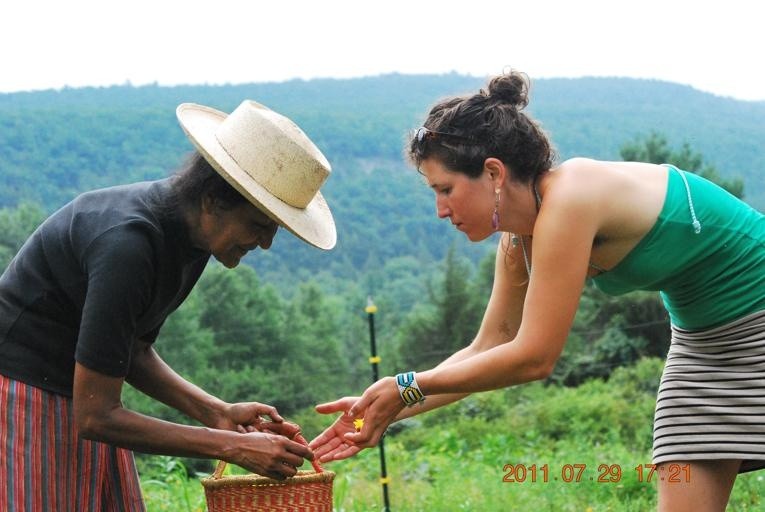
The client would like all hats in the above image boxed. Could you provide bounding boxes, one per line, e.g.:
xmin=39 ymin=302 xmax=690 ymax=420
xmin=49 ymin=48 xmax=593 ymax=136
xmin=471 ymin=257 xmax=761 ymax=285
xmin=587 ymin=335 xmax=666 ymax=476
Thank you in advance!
xmin=176 ymin=99 xmax=337 ymax=250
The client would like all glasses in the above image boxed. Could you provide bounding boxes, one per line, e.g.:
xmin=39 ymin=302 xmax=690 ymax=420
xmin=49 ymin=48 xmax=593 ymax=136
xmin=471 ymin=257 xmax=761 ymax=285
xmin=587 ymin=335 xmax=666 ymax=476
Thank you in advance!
xmin=414 ymin=127 xmax=481 ymax=145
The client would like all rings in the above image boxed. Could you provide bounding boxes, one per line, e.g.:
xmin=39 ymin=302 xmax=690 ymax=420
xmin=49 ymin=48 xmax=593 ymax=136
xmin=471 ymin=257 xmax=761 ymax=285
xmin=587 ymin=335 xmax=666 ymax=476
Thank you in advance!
xmin=342 ymin=441 xmax=350 ymax=450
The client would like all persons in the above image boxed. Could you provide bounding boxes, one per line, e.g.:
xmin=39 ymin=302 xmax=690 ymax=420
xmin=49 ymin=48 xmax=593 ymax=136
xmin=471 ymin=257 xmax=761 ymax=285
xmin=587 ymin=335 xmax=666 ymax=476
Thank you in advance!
xmin=0 ymin=98 xmax=339 ymax=512
xmin=305 ymin=68 xmax=765 ymax=511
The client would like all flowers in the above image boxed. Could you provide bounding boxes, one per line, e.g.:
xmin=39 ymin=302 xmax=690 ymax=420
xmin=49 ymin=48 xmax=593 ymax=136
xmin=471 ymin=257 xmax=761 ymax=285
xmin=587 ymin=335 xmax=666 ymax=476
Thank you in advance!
xmin=353 ymin=418 xmax=364 ymax=429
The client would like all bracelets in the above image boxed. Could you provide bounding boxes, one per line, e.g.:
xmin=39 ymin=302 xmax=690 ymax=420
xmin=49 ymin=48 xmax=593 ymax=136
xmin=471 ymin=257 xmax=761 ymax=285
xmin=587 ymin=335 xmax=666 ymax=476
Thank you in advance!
xmin=394 ymin=370 xmax=426 ymax=408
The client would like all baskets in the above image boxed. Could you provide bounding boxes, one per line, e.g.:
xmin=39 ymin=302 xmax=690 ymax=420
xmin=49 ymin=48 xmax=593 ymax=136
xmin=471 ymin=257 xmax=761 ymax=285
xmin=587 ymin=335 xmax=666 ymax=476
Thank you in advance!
xmin=199 ymin=421 xmax=337 ymax=512
